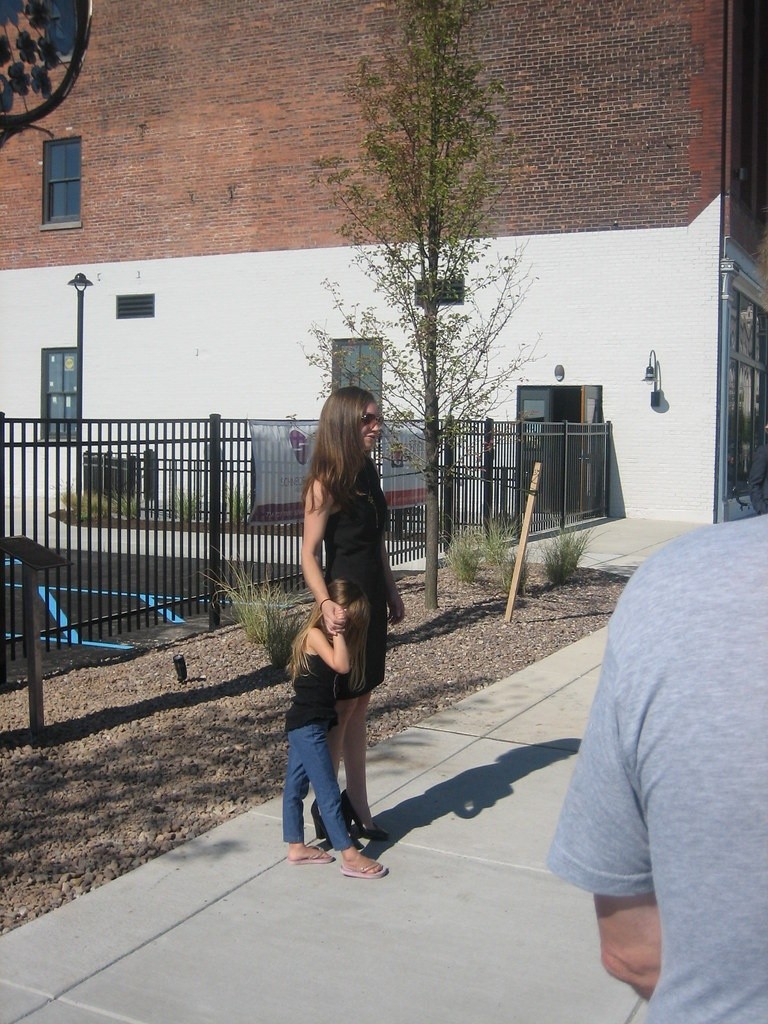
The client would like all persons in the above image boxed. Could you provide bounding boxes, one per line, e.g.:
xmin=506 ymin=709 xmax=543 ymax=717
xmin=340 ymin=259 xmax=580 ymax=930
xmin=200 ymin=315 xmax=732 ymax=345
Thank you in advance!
xmin=549 ymin=512 xmax=768 ymax=1024
xmin=301 ymin=386 xmax=404 ymax=850
xmin=748 ymin=442 xmax=768 ymax=515
xmin=282 ymin=579 xmax=390 ymax=879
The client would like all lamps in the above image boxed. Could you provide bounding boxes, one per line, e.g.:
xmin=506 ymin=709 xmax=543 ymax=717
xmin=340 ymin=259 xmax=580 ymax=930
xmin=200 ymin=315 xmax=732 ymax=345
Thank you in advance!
xmin=640 ymin=349 xmax=661 ymax=408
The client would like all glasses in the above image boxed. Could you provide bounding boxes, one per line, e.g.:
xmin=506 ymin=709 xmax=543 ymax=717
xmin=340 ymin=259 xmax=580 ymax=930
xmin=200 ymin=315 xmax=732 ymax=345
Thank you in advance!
xmin=362 ymin=413 xmax=384 ymax=426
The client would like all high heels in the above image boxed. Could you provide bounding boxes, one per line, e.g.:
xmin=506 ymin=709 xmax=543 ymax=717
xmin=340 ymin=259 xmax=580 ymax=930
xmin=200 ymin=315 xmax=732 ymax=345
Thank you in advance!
xmin=310 ymin=789 xmax=389 ymax=848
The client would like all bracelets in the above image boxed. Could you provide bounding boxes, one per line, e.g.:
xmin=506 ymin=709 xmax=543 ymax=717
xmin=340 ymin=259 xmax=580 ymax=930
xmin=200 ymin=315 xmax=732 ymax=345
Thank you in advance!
xmin=320 ymin=598 xmax=331 ymax=612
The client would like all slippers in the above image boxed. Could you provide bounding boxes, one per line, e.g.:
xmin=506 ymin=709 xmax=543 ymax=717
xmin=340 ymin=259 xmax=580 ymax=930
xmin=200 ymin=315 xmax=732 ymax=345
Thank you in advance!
xmin=340 ymin=859 xmax=387 ymax=879
xmin=289 ymin=845 xmax=333 ymax=864
